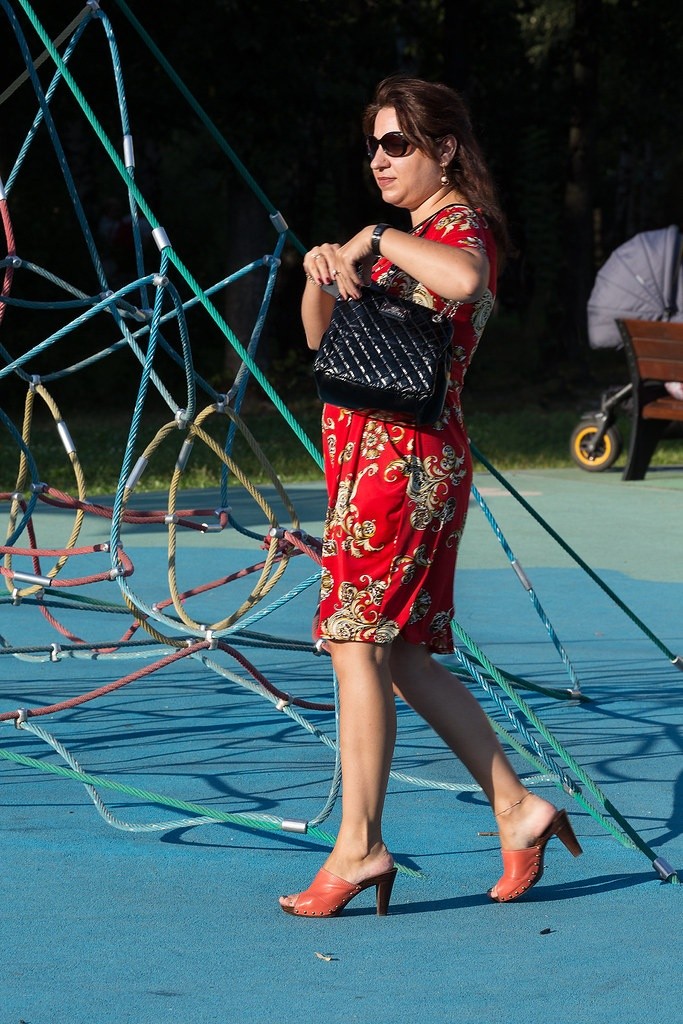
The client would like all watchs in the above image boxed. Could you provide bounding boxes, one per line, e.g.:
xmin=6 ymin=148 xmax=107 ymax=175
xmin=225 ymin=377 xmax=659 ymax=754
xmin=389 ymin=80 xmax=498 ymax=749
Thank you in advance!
xmin=371 ymin=224 xmax=393 ymax=258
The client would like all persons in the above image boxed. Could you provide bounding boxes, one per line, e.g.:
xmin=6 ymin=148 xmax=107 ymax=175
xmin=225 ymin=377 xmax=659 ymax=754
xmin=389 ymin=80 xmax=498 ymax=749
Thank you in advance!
xmin=277 ymin=80 xmax=581 ymax=916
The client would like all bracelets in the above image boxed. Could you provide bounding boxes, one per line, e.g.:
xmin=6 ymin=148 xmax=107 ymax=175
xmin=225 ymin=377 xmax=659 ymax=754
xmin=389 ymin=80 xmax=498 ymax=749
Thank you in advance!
xmin=306 ymin=272 xmax=322 ymax=287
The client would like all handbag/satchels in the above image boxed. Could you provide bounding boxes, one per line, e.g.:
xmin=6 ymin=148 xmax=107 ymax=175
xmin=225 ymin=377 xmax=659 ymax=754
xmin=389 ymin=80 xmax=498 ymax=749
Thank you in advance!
xmin=312 ymin=285 xmax=454 ymax=429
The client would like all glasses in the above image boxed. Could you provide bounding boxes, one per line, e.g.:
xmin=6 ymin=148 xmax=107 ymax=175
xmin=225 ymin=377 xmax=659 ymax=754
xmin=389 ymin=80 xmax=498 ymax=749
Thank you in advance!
xmin=365 ymin=130 xmax=431 ymax=160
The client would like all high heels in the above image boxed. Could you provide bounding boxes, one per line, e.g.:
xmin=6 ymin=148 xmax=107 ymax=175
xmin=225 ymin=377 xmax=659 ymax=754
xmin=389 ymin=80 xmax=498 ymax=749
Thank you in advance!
xmin=490 ymin=809 xmax=581 ymax=902
xmin=284 ymin=863 xmax=397 ymax=918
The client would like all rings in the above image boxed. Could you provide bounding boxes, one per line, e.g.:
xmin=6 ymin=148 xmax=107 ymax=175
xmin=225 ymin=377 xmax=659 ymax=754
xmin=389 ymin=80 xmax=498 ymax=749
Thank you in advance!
xmin=332 ymin=269 xmax=340 ymax=278
xmin=311 ymin=252 xmax=322 ymax=259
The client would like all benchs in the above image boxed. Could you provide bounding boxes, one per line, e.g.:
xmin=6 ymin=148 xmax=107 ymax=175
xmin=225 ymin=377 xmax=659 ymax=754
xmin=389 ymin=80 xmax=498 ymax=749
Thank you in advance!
xmin=614 ymin=318 xmax=683 ymax=481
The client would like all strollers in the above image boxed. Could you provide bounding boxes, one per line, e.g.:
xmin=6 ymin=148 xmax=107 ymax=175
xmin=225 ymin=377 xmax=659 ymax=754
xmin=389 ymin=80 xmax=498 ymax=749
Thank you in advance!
xmin=567 ymin=226 xmax=682 ymax=469
